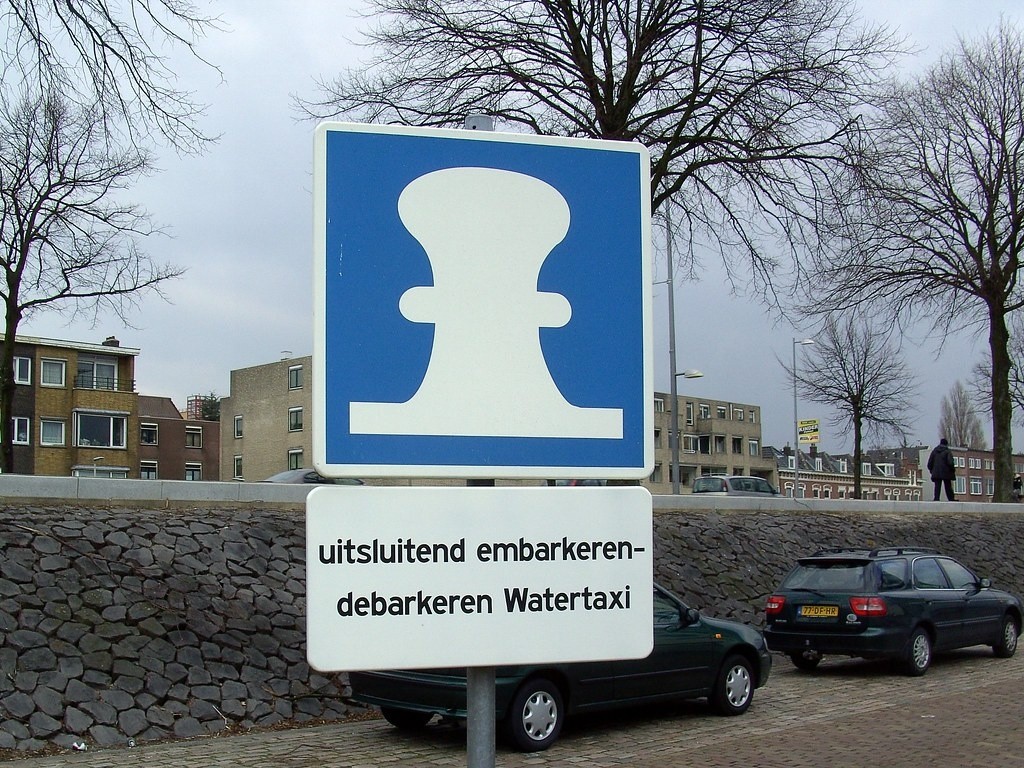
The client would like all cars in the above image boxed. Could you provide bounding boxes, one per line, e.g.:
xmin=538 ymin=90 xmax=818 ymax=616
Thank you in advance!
xmin=350 ymin=578 xmax=775 ymax=751
xmin=255 ymin=469 xmax=367 ymax=487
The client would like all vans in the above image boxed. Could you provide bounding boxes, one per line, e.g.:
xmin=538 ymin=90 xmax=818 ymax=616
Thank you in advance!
xmin=692 ymin=473 xmax=787 ymax=499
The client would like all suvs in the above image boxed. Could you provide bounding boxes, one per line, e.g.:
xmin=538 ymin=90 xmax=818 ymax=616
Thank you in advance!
xmin=763 ymin=545 xmax=1024 ymax=675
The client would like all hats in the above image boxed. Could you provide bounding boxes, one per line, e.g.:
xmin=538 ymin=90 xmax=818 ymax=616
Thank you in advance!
xmin=940 ymin=438 xmax=948 ymax=445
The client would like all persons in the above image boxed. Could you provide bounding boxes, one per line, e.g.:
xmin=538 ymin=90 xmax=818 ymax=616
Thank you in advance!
xmin=1013 ymin=473 xmax=1023 ymax=490
xmin=925 ymin=437 xmax=960 ymax=503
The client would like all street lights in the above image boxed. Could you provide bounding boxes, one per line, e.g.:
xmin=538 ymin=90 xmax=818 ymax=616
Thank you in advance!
xmin=791 ymin=338 xmax=817 ymax=498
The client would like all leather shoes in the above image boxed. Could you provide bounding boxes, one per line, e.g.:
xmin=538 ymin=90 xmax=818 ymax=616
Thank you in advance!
xmin=949 ymin=499 xmax=958 ymax=502
